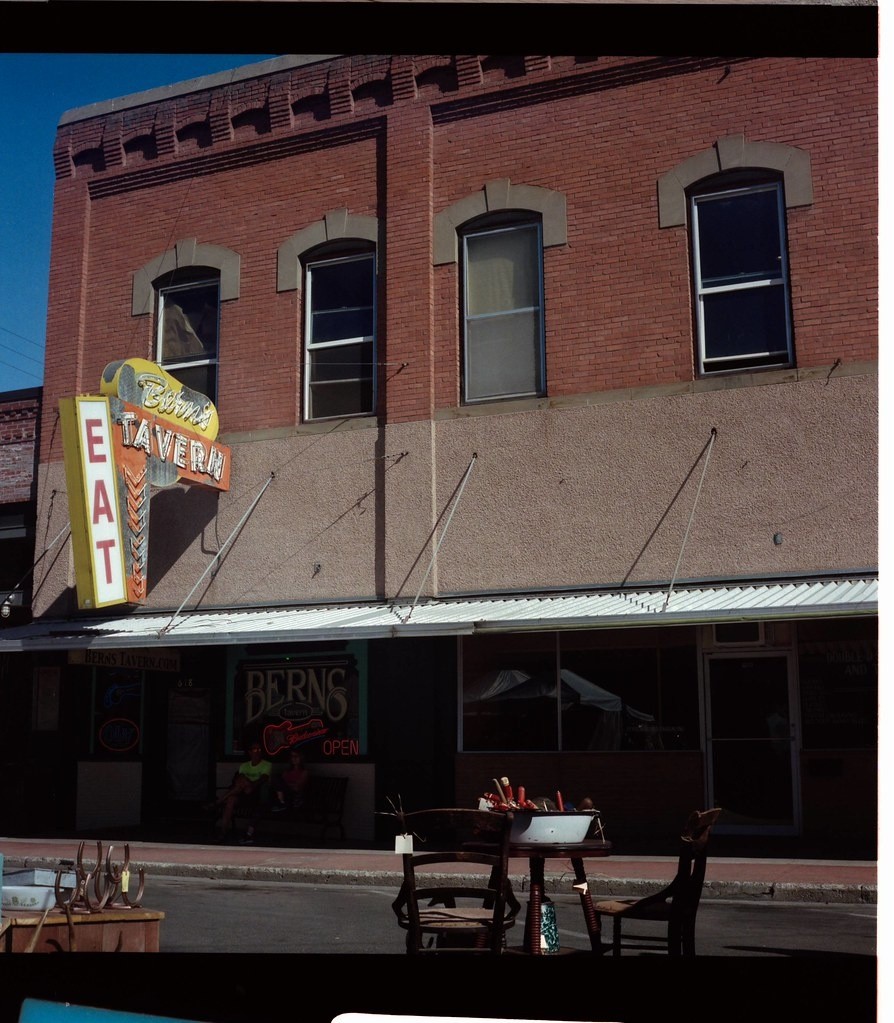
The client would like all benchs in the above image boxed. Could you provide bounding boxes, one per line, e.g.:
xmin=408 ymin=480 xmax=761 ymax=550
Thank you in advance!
xmin=203 ymin=774 xmax=349 ymax=843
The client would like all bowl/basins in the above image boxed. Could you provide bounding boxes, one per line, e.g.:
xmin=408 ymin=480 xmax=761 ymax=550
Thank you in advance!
xmin=1 ymin=885 xmax=64 ymax=911
xmin=402 ymin=808 xmax=507 ymax=844
xmin=494 ymin=810 xmax=596 ymax=843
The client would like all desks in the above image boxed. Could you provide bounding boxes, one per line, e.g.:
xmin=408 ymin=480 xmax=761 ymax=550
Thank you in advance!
xmin=0 ymin=902 xmax=165 ymax=951
xmin=448 ymin=836 xmax=612 ymax=956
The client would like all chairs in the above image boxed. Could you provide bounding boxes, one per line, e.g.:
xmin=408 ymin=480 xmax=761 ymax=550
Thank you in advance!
xmin=390 ymin=805 xmax=514 ymax=951
xmin=592 ymin=806 xmax=723 ymax=960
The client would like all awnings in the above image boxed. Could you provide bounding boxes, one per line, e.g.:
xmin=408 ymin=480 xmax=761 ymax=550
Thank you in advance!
xmin=1 ymin=433 xmax=878 ymax=655
